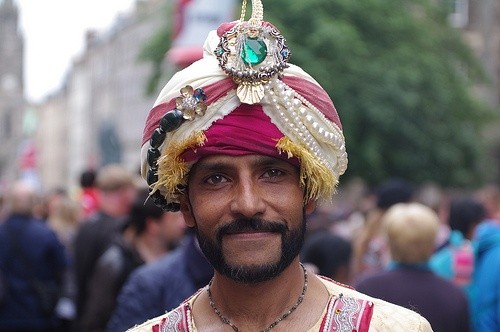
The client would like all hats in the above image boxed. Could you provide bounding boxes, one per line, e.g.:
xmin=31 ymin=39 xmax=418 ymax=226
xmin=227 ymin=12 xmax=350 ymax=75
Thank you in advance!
xmin=140 ymin=0 xmax=349 ymax=208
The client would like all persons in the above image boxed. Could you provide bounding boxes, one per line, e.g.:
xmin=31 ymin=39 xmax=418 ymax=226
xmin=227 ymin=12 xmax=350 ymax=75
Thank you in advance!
xmin=122 ymin=1 xmax=433 ymax=332
xmin=0 ymin=162 xmax=500 ymax=332
